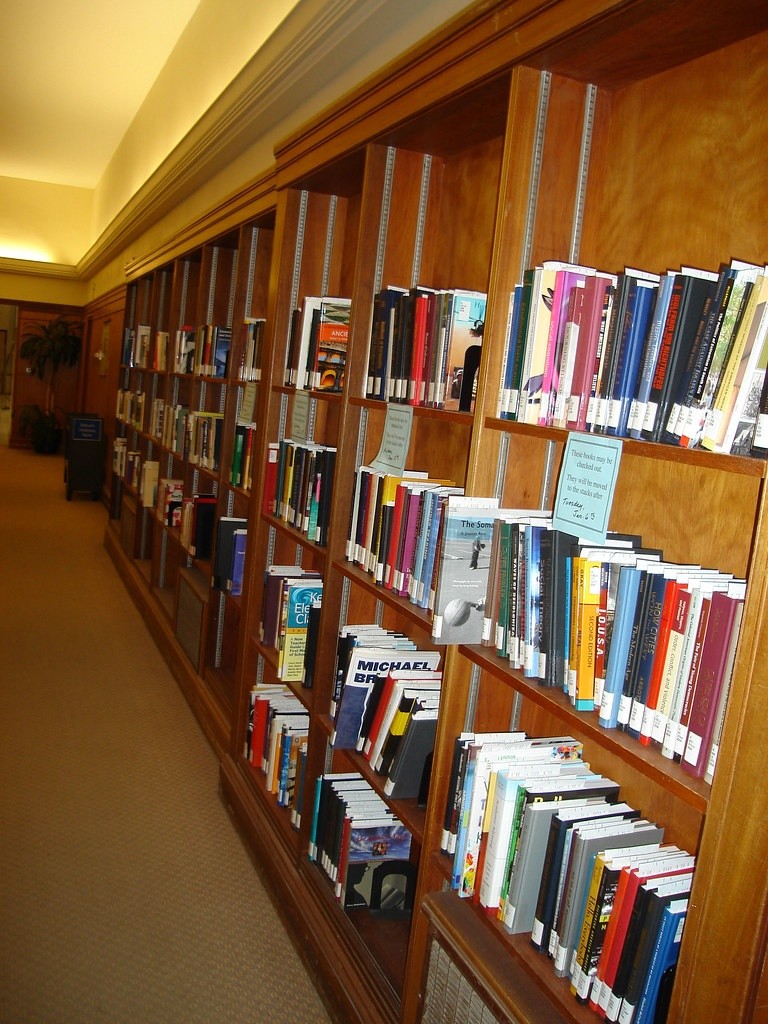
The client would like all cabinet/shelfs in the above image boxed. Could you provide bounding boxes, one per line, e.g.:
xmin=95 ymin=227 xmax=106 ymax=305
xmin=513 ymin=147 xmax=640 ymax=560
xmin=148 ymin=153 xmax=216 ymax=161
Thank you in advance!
xmin=103 ymin=0 xmax=768 ymax=1024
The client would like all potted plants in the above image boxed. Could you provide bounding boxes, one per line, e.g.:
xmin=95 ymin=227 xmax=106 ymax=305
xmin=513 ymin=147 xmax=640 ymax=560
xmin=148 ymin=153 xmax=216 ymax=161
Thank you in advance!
xmin=20 ymin=312 xmax=84 ymax=455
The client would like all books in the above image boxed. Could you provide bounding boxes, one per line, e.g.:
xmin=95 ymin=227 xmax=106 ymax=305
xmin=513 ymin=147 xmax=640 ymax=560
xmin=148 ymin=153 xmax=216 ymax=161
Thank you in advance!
xmin=283 ymin=296 xmax=352 ymax=392
xmin=365 ymin=285 xmax=487 ymax=413
xmin=211 ymin=516 xmax=248 ymax=594
xmin=243 ymin=684 xmax=310 ymax=832
xmin=261 ymin=438 xmax=337 ymax=547
xmin=440 ymin=732 xmax=697 ymax=1024
xmin=496 ymin=259 xmax=768 ymax=459
xmin=112 ymin=434 xmax=218 ymax=561
xmin=431 ymin=495 xmax=747 ymax=786
xmin=229 ymin=420 xmax=256 ymax=491
xmin=307 ymin=772 xmax=412 ymax=911
xmin=259 ymin=566 xmax=323 ymax=689
xmin=116 ymin=389 xmax=224 ymax=471
xmin=121 ymin=317 xmax=266 ymax=381
xmin=344 ymin=465 xmax=465 ymax=616
xmin=328 ymin=625 xmax=443 ymax=800
